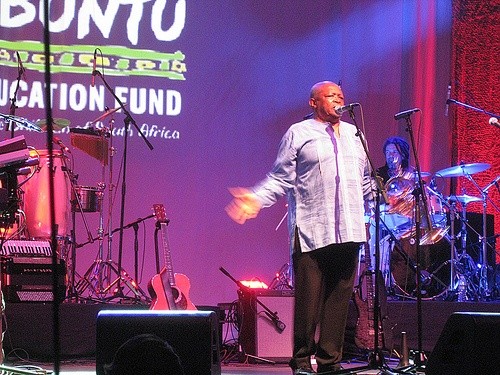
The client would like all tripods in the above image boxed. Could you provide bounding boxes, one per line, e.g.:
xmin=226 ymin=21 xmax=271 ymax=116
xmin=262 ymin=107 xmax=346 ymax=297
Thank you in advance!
xmin=316 ymin=110 xmax=500 ymax=375
xmin=218 ymin=267 xmax=280 ymax=365
xmin=60 ymin=72 xmax=156 ymax=305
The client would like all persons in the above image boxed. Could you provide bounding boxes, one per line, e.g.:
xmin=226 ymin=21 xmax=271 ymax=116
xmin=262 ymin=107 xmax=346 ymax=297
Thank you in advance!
xmin=225 ymin=80 xmax=379 ymax=375
xmin=371 ymin=137 xmax=415 ymax=205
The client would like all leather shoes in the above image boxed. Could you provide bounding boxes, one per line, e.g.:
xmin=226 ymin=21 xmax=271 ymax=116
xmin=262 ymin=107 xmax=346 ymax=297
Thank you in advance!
xmin=317 ymin=366 xmax=357 ymax=375
xmin=292 ymin=367 xmax=317 ymax=375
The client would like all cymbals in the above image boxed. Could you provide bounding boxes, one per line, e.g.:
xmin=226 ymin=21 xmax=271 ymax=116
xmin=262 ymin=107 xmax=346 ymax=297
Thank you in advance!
xmin=449 ymin=195 xmax=484 ymax=203
xmin=394 ymin=171 xmax=432 ymax=179
xmin=435 ymin=163 xmax=491 ymax=178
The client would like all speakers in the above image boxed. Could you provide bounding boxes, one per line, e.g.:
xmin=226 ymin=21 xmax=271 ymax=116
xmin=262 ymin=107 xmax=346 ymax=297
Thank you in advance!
xmin=238 ymin=288 xmax=295 ymax=364
xmin=95 ymin=309 xmax=221 ymax=375
xmin=428 ymin=311 xmax=500 ymax=375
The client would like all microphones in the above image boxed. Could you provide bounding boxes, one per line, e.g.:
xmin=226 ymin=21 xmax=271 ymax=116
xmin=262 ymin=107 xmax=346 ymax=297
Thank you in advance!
xmin=266 ymin=313 xmax=287 ymax=333
xmin=92 ymin=49 xmax=97 ymax=87
xmin=52 ymin=136 xmax=71 ymax=153
xmin=428 ymin=176 xmax=435 ymax=195
xmin=335 ymin=103 xmax=360 ymax=115
xmin=17 ymin=51 xmax=29 ymax=82
xmin=484 ymin=176 xmax=500 ymax=193
xmin=445 ymin=81 xmax=451 ymax=116
xmin=394 ymin=108 xmax=419 ymax=120
xmin=489 ymin=117 xmax=500 ymax=129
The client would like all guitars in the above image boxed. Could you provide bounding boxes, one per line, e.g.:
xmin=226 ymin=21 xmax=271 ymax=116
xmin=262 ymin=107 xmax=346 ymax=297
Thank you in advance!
xmin=146 ymin=203 xmax=198 ymax=311
xmin=352 ymin=222 xmax=386 ymax=352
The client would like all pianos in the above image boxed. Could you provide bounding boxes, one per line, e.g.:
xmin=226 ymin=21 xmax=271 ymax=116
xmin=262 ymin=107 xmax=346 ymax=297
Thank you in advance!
xmin=0 ymin=237 xmax=57 ymax=264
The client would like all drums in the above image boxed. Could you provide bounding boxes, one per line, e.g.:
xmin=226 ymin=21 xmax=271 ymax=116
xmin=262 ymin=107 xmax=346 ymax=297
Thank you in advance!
xmin=10 ymin=147 xmax=70 ymax=251
xmin=388 ymin=226 xmax=459 ymax=301
xmin=71 ymin=185 xmax=102 ymax=212
